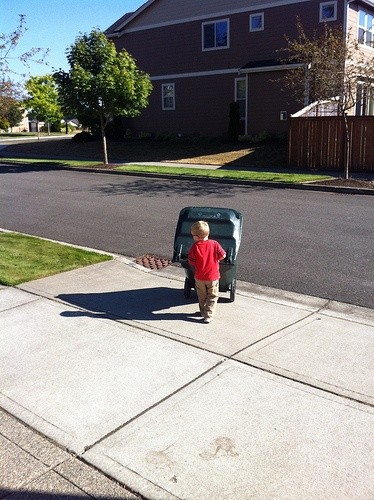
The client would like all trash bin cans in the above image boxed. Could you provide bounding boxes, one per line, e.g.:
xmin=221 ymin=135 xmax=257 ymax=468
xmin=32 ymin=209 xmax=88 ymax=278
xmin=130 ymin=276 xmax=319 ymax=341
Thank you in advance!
xmin=172 ymin=206 xmax=244 ymax=302
